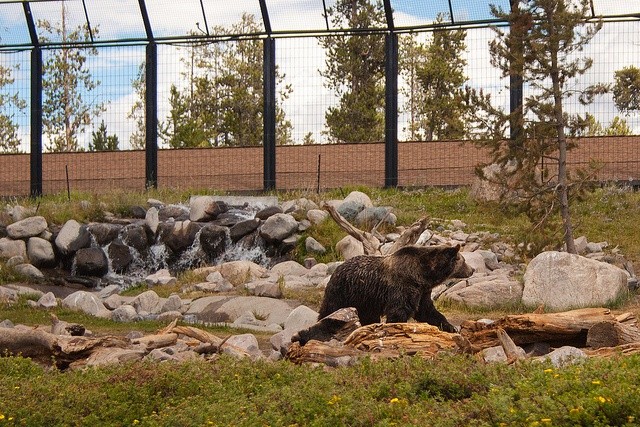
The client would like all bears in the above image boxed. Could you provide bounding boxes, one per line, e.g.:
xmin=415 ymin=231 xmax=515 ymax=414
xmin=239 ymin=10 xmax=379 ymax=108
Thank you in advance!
xmin=317 ymin=244 xmax=475 ymax=332
